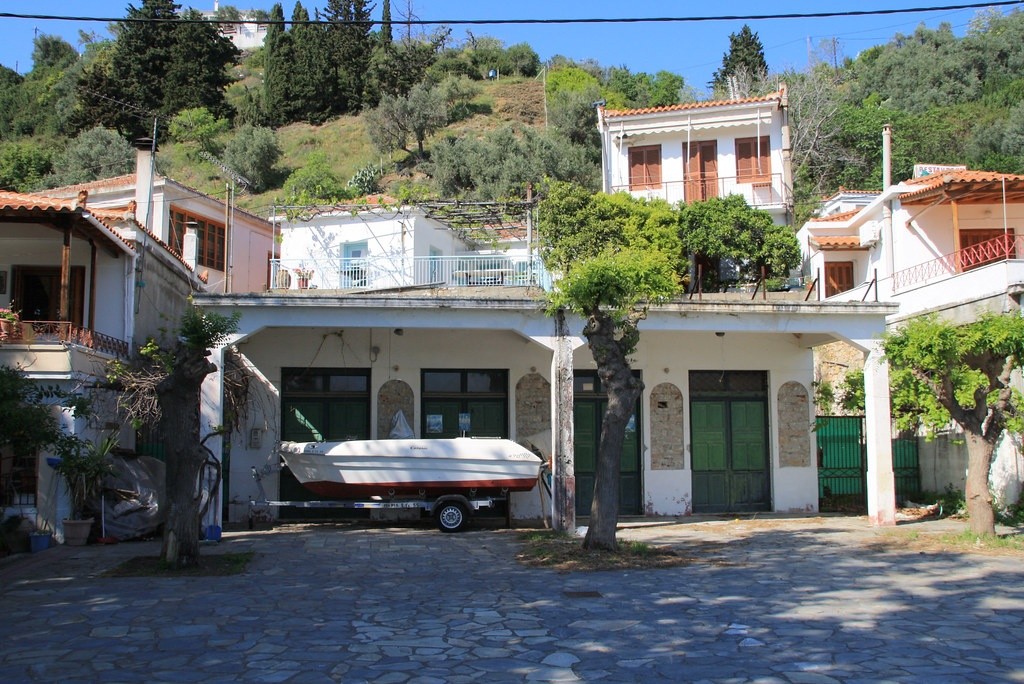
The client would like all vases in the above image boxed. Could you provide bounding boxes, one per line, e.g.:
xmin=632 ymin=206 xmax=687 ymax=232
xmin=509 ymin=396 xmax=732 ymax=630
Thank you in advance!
xmin=0 ymin=320 xmax=12 ymax=334
xmin=298 ymin=278 xmax=309 ymax=289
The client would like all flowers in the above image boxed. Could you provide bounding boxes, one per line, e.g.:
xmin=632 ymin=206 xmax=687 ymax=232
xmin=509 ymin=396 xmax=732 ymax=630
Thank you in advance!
xmin=0 ymin=299 xmax=22 ymax=321
xmin=293 ymin=268 xmax=314 ymax=281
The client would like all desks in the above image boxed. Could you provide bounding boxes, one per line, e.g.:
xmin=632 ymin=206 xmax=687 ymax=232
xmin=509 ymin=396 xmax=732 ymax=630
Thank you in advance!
xmin=453 ymin=269 xmax=497 ymax=285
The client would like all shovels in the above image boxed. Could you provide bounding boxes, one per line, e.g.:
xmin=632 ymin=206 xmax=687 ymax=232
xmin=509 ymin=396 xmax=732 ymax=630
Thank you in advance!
xmin=204 ymin=488 xmax=222 ymax=542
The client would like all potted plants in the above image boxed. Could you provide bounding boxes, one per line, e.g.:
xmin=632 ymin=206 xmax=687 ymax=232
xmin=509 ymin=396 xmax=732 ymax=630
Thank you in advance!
xmin=47 ymin=430 xmax=120 ymax=546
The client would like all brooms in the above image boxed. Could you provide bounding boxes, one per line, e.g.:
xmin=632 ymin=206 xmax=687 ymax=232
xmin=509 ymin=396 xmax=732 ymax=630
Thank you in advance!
xmin=38 ymin=456 xmax=65 ymax=532
xmin=97 ymin=495 xmax=119 ymax=545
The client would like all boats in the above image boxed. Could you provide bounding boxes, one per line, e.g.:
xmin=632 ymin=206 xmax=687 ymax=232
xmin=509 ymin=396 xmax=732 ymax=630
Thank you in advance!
xmin=279 ymin=440 xmax=544 ymax=496
